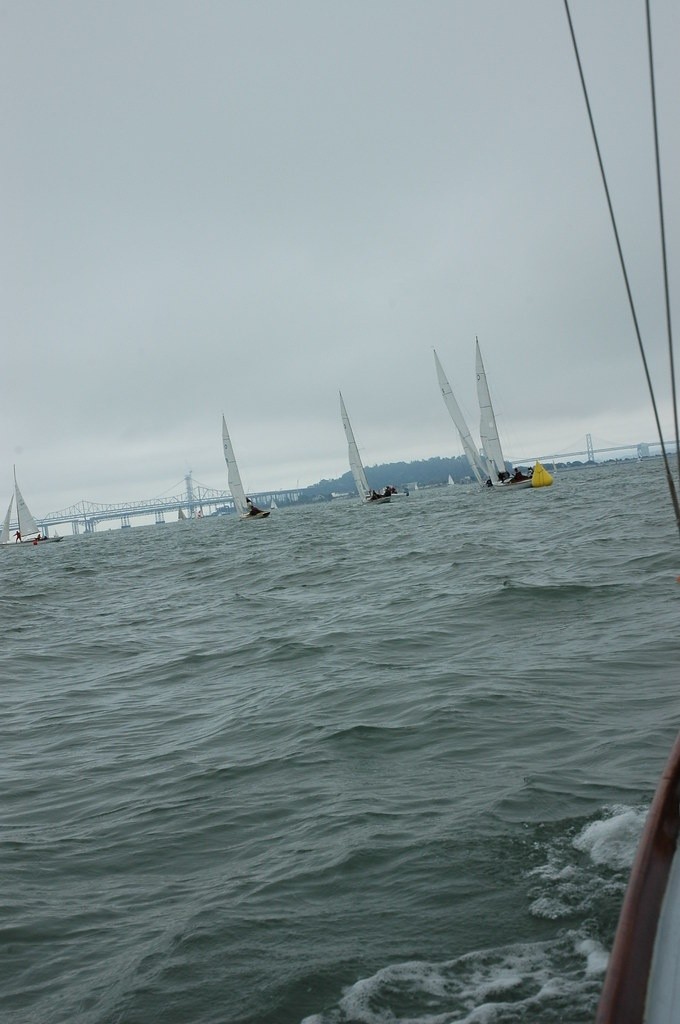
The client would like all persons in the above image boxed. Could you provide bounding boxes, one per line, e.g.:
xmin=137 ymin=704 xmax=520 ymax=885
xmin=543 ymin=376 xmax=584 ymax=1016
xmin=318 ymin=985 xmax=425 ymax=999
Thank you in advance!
xmin=384 ymin=487 xmax=392 ymax=497
xmin=38 ymin=534 xmax=41 ymax=539
xmin=249 ymin=506 xmax=261 ymax=516
xmin=507 ymin=468 xmax=529 ymax=483
xmin=13 ymin=531 xmax=22 ymax=544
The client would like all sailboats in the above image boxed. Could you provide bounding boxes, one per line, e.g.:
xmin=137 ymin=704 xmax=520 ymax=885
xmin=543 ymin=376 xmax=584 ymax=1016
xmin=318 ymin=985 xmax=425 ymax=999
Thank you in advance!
xmin=472 ymin=335 xmax=555 ymax=492
xmin=433 ymin=348 xmax=502 ymax=493
xmin=446 ymin=473 xmax=456 ymax=487
xmin=0 ymin=464 xmax=66 ymax=549
xmin=220 ymin=413 xmax=274 ymax=523
xmin=337 ymin=388 xmax=396 ymax=506
xmin=269 ymin=498 xmax=278 ymax=509
